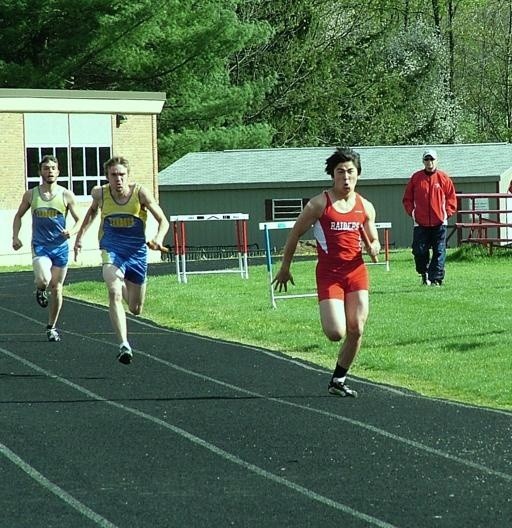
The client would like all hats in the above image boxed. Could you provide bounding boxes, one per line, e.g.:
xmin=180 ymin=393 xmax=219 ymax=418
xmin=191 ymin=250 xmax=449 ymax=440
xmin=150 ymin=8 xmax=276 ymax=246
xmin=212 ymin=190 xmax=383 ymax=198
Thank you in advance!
xmin=422 ymin=149 xmax=437 ymax=159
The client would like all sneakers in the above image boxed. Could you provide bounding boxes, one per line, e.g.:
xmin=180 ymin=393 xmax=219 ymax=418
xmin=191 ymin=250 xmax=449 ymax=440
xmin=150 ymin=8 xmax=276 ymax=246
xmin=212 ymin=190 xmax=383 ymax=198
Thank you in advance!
xmin=46 ymin=326 xmax=62 ymax=341
xmin=116 ymin=345 xmax=134 ymax=365
xmin=327 ymin=380 xmax=358 ymax=398
xmin=421 ymin=272 xmax=431 ymax=285
xmin=36 ymin=287 xmax=49 ymax=308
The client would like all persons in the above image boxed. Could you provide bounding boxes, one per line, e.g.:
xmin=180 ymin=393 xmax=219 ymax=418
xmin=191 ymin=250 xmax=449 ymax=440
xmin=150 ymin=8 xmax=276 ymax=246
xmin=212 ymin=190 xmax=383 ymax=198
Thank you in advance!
xmin=72 ymin=154 xmax=172 ymax=365
xmin=11 ymin=154 xmax=88 ymax=343
xmin=268 ymin=145 xmax=382 ymax=400
xmin=401 ymin=148 xmax=458 ymax=287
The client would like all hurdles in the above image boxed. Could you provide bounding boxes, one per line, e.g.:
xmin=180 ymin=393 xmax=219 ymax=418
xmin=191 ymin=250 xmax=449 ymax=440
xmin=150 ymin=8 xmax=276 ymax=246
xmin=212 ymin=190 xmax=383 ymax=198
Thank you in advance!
xmin=258 ymin=222 xmax=318 ymax=307
xmin=171 ymin=213 xmax=250 ymax=283
xmin=358 ymin=223 xmax=392 ymax=271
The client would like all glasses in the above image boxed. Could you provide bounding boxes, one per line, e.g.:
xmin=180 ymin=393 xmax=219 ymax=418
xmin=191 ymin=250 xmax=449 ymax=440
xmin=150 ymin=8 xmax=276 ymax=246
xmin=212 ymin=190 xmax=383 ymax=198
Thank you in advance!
xmin=424 ymin=158 xmax=435 ymax=161
